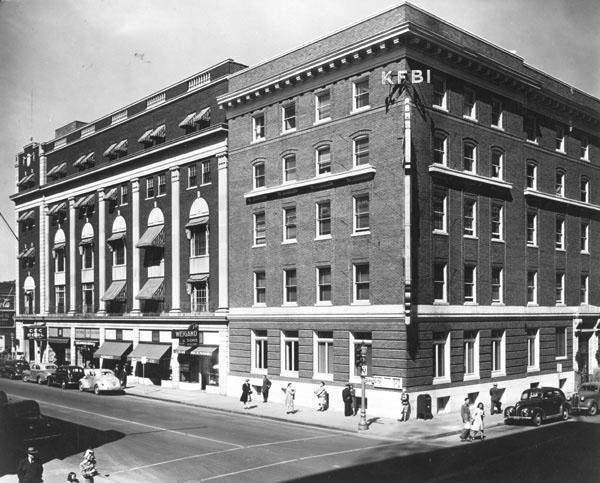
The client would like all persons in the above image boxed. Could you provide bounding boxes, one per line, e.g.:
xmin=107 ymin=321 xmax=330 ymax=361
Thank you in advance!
xmin=397 ymin=388 xmax=410 ymax=422
xmin=114 ymin=364 xmax=127 ymax=389
xmin=67 ymin=472 xmax=80 ymax=483
xmin=240 ymin=379 xmax=253 ymax=409
xmin=17 ymin=447 xmax=43 ymax=483
xmin=285 ymin=383 xmax=295 ymax=413
xmin=314 ymin=381 xmax=328 ymax=411
xmin=489 ymin=383 xmax=503 ymax=415
xmin=261 ymin=374 xmax=272 ymax=403
xmin=460 ymin=398 xmax=485 ymax=441
xmin=80 ymin=450 xmax=97 ymax=483
xmin=342 ymin=384 xmax=356 ymax=416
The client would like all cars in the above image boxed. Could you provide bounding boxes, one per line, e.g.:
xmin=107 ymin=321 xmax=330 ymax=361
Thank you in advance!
xmin=0 ymin=359 xmax=122 ymax=395
xmin=0 ymin=401 xmax=62 ymax=446
xmin=504 ymin=382 xmax=600 ymax=425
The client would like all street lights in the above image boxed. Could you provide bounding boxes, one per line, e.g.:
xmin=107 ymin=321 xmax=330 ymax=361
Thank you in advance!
xmin=360 ymin=341 xmax=367 ymax=428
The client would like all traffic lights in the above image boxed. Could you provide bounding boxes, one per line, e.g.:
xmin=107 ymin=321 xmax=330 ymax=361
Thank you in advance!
xmin=354 ymin=343 xmax=363 ymax=367
